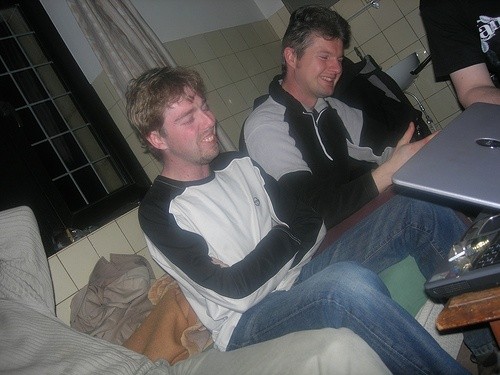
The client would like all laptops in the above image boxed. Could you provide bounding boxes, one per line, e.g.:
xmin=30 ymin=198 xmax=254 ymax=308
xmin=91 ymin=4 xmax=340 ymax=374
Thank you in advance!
xmin=391 ymin=101 xmax=500 ymax=299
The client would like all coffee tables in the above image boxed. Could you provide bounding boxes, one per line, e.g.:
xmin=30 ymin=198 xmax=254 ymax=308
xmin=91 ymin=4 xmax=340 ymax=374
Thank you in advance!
xmin=436 ymin=288 xmax=500 ymax=353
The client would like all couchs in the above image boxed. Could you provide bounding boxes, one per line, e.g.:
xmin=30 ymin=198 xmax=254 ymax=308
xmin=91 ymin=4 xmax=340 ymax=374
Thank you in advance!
xmin=378 ymin=225 xmax=464 ymax=364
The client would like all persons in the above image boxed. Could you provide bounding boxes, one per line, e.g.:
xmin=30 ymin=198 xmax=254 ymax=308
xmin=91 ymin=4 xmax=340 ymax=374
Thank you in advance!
xmin=125 ymin=66 xmax=500 ymax=375
xmin=239 ymin=4 xmax=440 ymax=233
xmin=420 ymin=1 xmax=500 ymax=110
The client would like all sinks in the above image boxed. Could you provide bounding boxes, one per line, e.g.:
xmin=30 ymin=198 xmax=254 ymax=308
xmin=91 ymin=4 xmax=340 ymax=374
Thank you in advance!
xmin=384 ymin=51 xmax=421 ymax=91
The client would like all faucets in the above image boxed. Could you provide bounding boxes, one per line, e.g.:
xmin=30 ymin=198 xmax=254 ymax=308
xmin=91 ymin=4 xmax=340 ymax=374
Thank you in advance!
xmin=353 ymin=46 xmax=365 ymax=61
